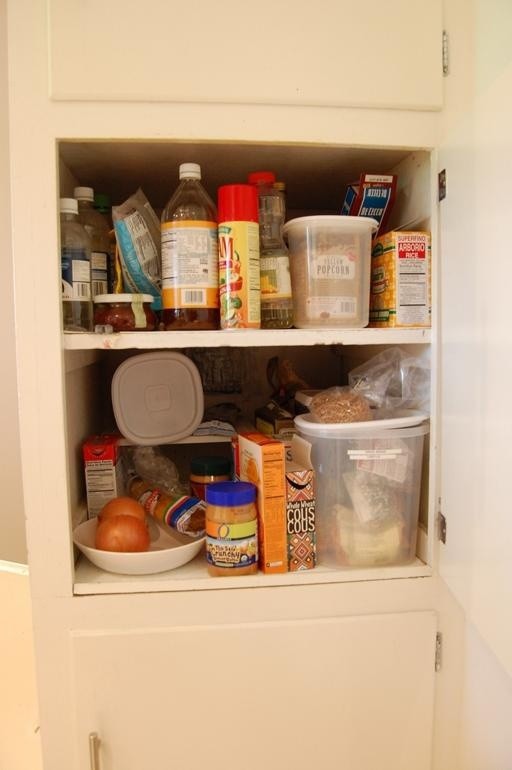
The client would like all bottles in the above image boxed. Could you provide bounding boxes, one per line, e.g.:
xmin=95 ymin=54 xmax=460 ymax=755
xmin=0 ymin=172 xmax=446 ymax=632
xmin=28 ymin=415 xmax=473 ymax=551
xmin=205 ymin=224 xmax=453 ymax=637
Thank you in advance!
xmin=162 ymin=162 xmax=293 ymax=329
xmin=60 ymin=184 xmax=109 ymax=332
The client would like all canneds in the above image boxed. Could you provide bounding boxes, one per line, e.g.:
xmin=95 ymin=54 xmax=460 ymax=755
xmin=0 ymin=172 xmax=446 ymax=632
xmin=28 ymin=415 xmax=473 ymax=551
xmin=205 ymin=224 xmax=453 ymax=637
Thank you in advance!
xmin=189 ymin=454 xmax=231 ymax=501
xmin=93 ymin=293 xmax=158 ymax=332
xmin=205 ymin=481 xmax=259 ymax=578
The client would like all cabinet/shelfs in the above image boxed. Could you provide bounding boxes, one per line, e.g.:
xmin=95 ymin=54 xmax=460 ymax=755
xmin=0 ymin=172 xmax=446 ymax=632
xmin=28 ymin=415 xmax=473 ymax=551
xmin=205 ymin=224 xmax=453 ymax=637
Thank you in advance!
xmin=4 ymin=3 xmax=509 ymax=765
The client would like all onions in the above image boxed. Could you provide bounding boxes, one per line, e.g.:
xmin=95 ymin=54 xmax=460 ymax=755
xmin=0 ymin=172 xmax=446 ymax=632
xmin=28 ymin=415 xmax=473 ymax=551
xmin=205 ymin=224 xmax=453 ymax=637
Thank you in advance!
xmin=96 ymin=497 xmax=151 ymax=553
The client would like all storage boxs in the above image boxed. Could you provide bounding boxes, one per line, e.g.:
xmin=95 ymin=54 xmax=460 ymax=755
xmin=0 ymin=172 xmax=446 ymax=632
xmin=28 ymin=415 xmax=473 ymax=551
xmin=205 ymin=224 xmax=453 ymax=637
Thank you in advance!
xmin=294 ymin=408 xmax=428 ymax=570
xmin=284 ymin=214 xmax=380 ymax=330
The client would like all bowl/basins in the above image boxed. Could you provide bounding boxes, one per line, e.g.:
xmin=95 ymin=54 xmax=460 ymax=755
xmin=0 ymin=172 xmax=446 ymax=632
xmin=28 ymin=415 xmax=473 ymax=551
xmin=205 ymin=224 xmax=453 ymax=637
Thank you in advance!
xmin=73 ymin=512 xmax=207 ymax=578
